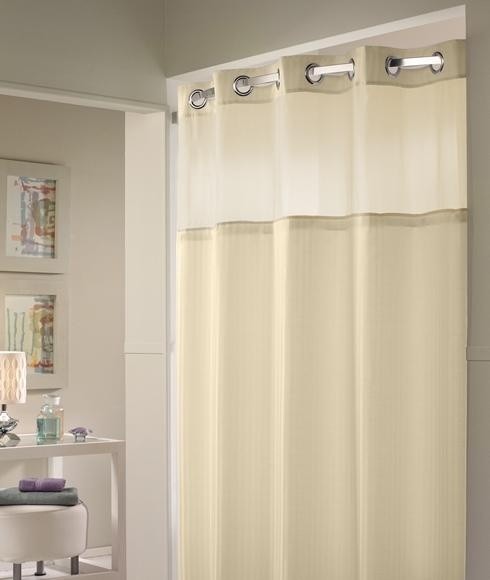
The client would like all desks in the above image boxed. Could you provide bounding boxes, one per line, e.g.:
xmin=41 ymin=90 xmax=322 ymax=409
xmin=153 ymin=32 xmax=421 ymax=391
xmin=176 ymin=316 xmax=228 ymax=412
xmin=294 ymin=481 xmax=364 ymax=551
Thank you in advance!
xmin=0 ymin=430 xmax=125 ymax=580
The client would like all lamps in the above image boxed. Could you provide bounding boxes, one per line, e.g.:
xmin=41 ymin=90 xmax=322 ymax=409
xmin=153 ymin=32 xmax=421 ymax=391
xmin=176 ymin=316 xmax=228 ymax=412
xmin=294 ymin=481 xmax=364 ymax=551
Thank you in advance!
xmin=0 ymin=352 xmax=26 ymax=433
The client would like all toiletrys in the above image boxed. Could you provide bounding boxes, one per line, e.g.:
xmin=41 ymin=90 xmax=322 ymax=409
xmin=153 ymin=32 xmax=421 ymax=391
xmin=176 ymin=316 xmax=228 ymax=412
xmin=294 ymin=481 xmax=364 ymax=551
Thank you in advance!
xmin=36 ymin=403 xmax=61 ymax=446
xmin=44 ymin=392 xmax=66 ymax=441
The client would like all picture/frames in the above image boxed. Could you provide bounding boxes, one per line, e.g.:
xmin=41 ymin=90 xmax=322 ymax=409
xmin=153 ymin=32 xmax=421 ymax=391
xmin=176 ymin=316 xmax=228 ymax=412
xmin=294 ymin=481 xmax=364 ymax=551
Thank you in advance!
xmin=0 ymin=155 xmax=74 ymax=392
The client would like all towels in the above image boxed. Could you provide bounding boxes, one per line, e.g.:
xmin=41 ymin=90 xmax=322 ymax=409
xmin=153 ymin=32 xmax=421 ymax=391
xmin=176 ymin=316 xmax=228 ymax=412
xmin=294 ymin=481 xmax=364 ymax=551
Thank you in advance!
xmin=19 ymin=476 xmax=68 ymax=493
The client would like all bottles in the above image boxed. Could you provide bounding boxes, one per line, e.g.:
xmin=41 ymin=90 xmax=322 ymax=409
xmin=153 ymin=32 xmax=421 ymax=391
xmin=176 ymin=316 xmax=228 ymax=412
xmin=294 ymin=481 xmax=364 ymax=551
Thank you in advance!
xmin=33 ymin=393 xmax=60 ymax=444
xmin=50 ymin=395 xmax=66 ymax=442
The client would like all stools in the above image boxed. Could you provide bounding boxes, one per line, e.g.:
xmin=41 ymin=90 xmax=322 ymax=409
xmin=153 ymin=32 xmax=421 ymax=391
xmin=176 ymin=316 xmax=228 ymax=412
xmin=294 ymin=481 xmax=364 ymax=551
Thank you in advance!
xmin=3 ymin=504 xmax=90 ymax=580
